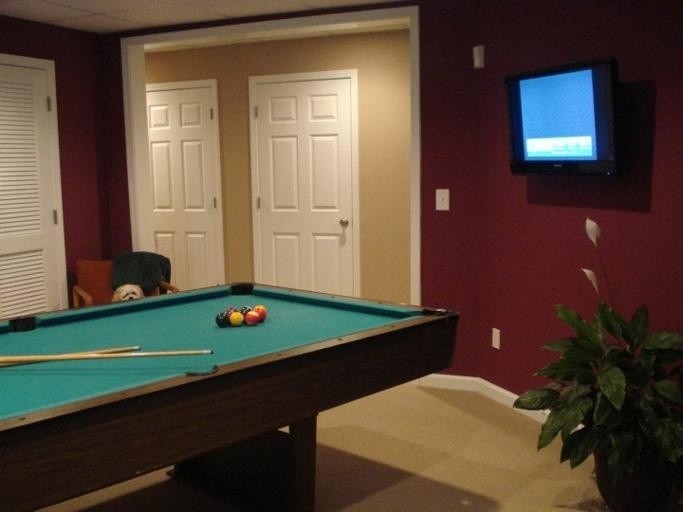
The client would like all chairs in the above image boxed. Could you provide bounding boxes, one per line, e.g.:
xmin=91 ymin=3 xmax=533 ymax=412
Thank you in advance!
xmin=72 ymin=259 xmax=180 ymax=310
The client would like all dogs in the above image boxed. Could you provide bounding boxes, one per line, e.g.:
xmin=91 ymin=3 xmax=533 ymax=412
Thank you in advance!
xmin=112 ymin=284 xmax=145 ymax=303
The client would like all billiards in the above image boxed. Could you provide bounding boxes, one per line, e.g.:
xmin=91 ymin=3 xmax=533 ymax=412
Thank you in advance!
xmin=215 ymin=305 xmax=266 ymax=327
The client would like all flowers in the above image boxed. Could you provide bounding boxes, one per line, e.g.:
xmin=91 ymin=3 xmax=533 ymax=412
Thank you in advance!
xmin=512 ymin=217 xmax=683 ymax=481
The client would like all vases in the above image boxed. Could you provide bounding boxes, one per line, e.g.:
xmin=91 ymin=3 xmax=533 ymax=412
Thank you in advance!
xmin=593 ymin=449 xmax=683 ymax=512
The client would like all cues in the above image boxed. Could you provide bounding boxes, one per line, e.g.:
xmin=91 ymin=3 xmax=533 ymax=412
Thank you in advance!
xmin=0 ymin=351 xmax=213 ymax=363
xmin=0 ymin=346 xmax=141 ymax=367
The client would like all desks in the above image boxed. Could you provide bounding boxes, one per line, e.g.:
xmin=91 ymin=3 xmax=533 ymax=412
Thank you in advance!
xmin=1 ymin=283 xmax=462 ymax=512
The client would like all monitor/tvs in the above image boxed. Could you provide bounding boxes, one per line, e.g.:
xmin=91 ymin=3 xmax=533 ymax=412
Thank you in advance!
xmin=505 ymin=55 xmax=623 ymax=177
